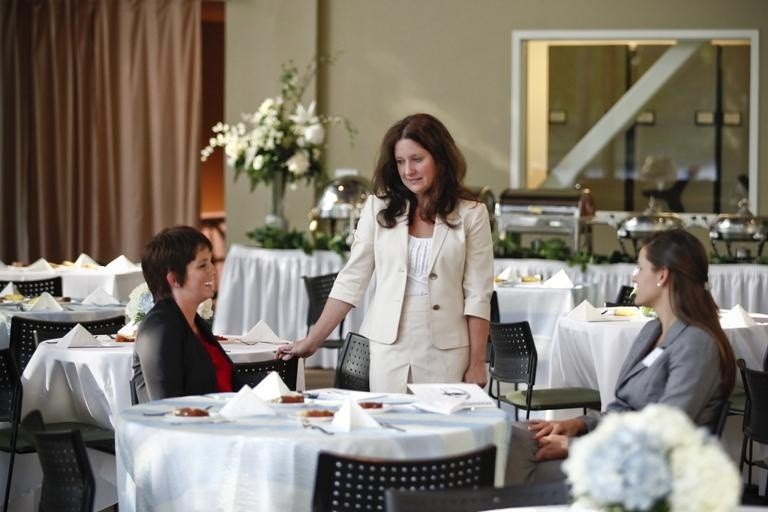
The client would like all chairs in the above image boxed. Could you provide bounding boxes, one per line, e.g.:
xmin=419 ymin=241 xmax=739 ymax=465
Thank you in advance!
xmin=0 ymin=215 xmax=767 ymax=511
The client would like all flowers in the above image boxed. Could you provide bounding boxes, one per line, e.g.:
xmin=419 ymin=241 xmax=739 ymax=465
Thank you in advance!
xmin=195 ymin=59 xmax=355 ymax=209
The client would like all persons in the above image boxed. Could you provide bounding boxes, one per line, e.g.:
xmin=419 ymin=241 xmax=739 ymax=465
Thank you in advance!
xmin=132 ymin=226 xmax=240 ymax=404
xmin=274 ymin=113 xmax=492 ymax=394
xmin=505 ymin=227 xmax=737 ymax=489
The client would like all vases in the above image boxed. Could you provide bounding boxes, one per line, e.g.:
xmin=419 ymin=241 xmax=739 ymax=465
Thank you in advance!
xmin=261 ymin=162 xmax=288 ymax=234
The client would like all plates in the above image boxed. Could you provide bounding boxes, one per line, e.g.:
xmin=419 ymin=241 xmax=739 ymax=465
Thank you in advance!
xmin=45 ymin=325 xmax=290 ymax=353
xmin=165 ymin=386 xmax=429 ymax=435
xmin=0 ymin=293 xmax=127 ymax=314
